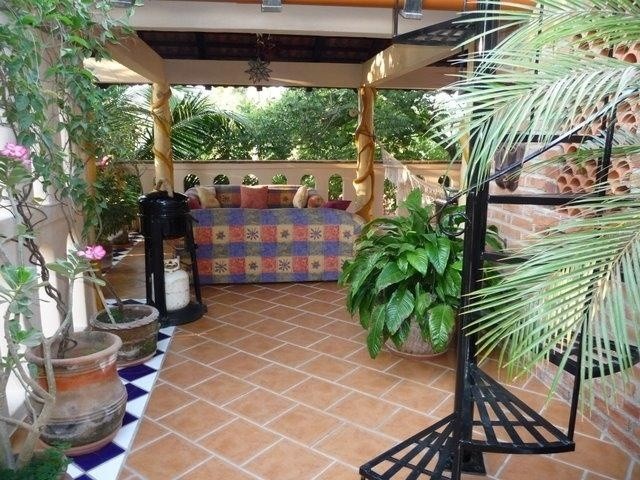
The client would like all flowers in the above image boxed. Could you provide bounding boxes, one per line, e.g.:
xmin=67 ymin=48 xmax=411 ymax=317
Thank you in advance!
xmin=0 ymin=244 xmax=105 ymax=470
xmin=0 ymin=143 xmax=105 ymax=359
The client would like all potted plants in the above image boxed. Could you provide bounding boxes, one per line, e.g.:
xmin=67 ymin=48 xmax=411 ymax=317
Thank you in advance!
xmin=91 ymin=172 xmax=144 ymax=258
xmin=0 ymin=0 xmax=159 ymax=367
xmin=0 ymin=0 xmax=127 ymax=456
xmin=339 ymin=188 xmax=507 ymax=360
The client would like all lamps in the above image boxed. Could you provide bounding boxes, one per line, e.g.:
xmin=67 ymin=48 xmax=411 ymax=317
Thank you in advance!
xmin=392 ymin=0 xmax=423 ymax=37
xmin=113 ymin=0 xmax=135 ymax=8
xmin=245 ymin=33 xmax=277 ymax=84
xmin=261 ymin=0 xmax=282 ymax=13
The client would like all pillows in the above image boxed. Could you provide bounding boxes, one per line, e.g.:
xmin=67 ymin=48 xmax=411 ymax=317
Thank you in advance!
xmin=195 ymin=186 xmax=220 ymax=208
xmin=318 ymin=201 xmax=353 ymax=210
xmin=239 ymin=186 xmax=269 ymax=208
xmin=293 ymin=185 xmax=309 ymax=209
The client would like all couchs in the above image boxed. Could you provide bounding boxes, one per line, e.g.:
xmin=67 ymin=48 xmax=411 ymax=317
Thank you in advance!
xmin=183 ymin=184 xmax=325 ymax=208
xmin=185 ymin=208 xmax=368 ymax=284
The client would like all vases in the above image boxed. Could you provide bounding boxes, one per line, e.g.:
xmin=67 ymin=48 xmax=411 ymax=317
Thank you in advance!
xmin=0 ymin=451 xmax=67 ymax=479
xmin=1 ymin=449 xmax=76 ymax=479
